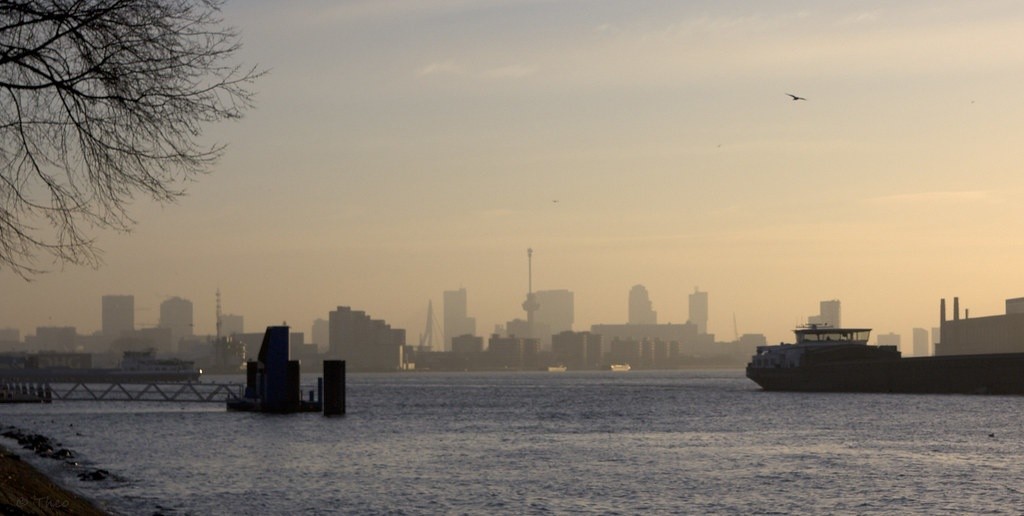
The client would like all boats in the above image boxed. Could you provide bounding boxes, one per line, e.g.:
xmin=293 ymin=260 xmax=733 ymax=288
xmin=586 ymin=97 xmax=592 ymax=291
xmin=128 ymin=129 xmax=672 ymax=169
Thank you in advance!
xmin=611 ymin=364 xmax=632 ymax=371
xmin=548 ymin=363 xmax=567 ymax=373
xmin=54 ymin=347 xmax=203 ymax=384
xmin=749 ymin=324 xmax=1024 ymax=396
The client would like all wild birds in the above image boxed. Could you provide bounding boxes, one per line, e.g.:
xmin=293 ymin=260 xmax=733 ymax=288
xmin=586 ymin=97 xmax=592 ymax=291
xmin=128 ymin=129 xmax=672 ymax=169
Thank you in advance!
xmin=787 ymin=93 xmax=806 ymax=101
